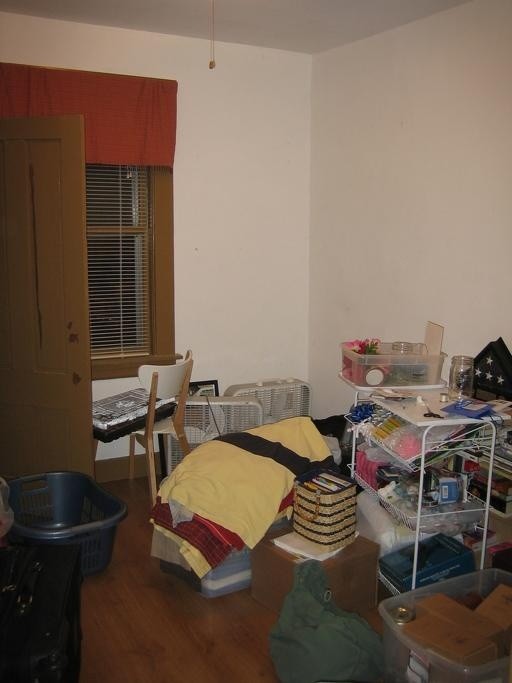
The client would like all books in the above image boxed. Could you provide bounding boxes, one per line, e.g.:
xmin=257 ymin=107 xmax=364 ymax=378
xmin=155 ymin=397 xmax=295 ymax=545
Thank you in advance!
xmin=445 ymin=438 xmax=511 ymax=550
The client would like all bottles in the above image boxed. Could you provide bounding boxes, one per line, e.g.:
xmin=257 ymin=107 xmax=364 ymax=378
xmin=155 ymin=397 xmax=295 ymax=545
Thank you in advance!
xmin=447 ymin=353 xmax=475 ymax=401
xmin=391 ymin=341 xmax=414 ymax=385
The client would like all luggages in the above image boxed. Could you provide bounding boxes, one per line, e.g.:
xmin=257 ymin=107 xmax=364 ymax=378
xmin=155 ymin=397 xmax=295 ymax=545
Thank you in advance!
xmin=0 ymin=539 xmax=81 ymax=682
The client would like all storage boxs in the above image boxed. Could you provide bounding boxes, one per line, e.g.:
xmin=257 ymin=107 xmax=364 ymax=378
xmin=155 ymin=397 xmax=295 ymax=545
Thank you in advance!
xmin=159 ymin=544 xmax=253 ymax=598
xmin=262 ymin=528 xmax=379 ymax=626
xmin=378 ymin=533 xmax=476 ymax=599
xmin=382 ymin=568 xmax=512 ymax=683
xmin=340 ymin=341 xmax=448 ymax=387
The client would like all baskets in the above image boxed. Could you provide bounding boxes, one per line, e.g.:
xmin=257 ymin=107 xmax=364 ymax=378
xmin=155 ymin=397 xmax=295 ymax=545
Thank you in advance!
xmin=10 ymin=471 xmax=129 ymax=578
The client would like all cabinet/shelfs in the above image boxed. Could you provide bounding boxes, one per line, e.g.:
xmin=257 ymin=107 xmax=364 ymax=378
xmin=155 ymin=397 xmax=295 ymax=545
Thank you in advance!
xmin=338 ymin=372 xmax=512 ymax=603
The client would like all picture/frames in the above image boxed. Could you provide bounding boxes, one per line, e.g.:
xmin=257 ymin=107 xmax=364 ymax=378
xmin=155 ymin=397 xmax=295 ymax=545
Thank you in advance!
xmin=158 ymin=379 xmax=219 ymax=479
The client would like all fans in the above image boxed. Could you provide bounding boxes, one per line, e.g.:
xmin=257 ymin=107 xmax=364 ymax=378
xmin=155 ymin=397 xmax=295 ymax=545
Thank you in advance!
xmin=223 ymin=378 xmax=311 ymax=436
xmin=165 ymin=396 xmax=263 ymax=477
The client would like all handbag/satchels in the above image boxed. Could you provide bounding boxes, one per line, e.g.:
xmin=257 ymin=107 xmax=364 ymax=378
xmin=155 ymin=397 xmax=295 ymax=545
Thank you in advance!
xmin=268 ymin=560 xmax=383 ymax=682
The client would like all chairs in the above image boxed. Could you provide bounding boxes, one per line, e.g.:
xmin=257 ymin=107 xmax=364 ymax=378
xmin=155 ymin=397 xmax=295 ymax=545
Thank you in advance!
xmin=94 ymin=350 xmax=194 ymax=509
